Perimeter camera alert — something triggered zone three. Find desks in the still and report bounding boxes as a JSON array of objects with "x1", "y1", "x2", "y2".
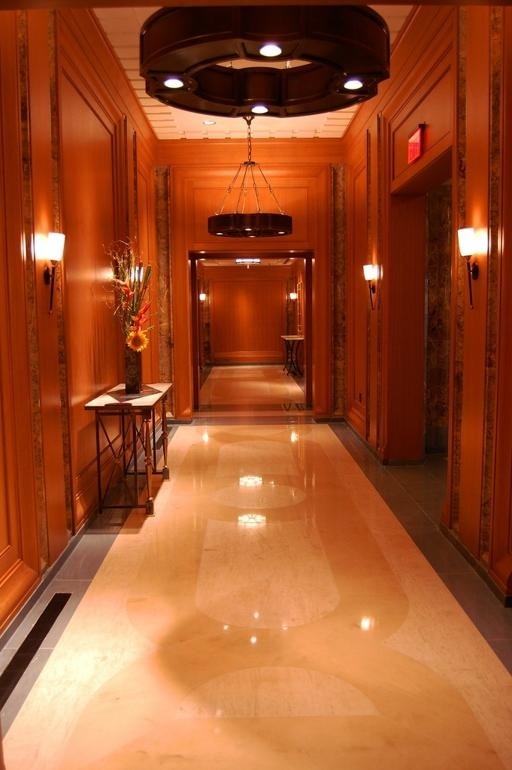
[
  {"x1": 81, "y1": 378, "x2": 176, "y2": 518},
  {"x1": 279, "y1": 334, "x2": 306, "y2": 382}
]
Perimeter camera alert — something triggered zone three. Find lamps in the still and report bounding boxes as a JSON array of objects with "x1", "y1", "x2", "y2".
[
  {"x1": 289, "y1": 291, "x2": 297, "y2": 302},
  {"x1": 452, "y1": 225, "x2": 481, "y2": 309},
  {"x1": 39, "y1": 231, "x2": 67, "y2": 313},
  {"x1": 137, "y1": 3, "x2": 393, "y2": 123},
  {"x1": 361, "y1": 261, "x2": 374, "y2": 305},
  {"x1": 199, "y1": 294, "x2": 207, "y2": 301},
  {"x1": 203, "y1": 111, "x2": 293, "y2": 243}
]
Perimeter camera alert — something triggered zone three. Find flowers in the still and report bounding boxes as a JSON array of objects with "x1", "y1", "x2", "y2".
[{"x1": 104, "y1": 233, "x2": 169, "y2": 353}]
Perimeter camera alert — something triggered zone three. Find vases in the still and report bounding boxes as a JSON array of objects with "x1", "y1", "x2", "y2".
[{"x1": 125, "y1": 347, "x2": 141, "y2": 394}]
